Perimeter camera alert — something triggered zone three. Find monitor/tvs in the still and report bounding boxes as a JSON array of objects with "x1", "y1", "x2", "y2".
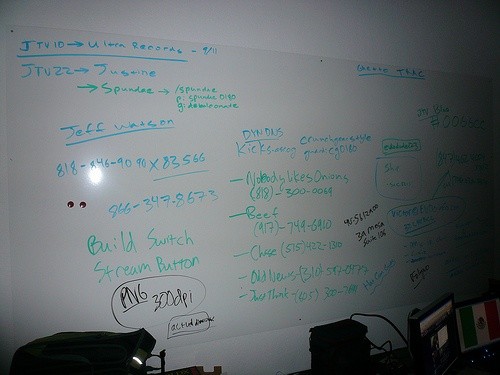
[{"x1": 408, "y1": 292, "x2": 461, "y2": 375}]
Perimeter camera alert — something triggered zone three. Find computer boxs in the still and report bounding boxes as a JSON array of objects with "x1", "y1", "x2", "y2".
[{"x1": 309, "y1": 318, "x2": 370, "y2": 375}]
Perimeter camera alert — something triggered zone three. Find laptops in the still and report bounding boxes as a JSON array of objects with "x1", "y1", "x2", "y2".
[{"x1": 453, "y1": 295, "x2": 500, "y2": 375}]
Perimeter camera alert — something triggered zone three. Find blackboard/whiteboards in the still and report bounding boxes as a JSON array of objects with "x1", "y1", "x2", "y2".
[{"x1": 0, "y1": 24, "x2": 500, "y2": 374}]
